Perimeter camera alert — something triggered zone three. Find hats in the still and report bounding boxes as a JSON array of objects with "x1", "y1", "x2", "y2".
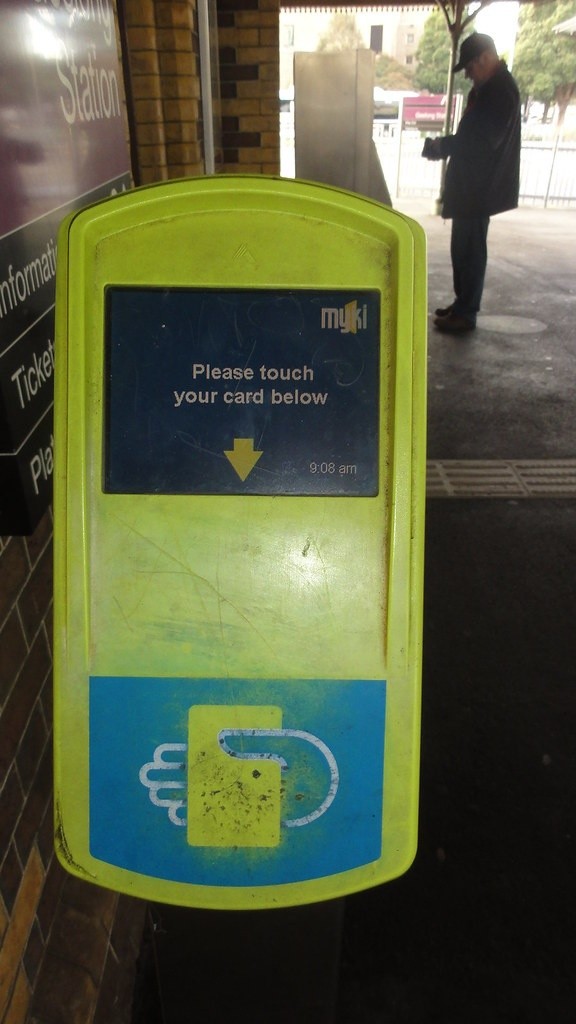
[{"x1": 453, "y1": 33, "x2": 494, "y2": 73}]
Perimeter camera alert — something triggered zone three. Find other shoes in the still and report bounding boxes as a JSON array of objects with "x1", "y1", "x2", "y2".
[
  {"x1": 435, "y1": 311, "x2": 476, "y2": 331},
  {"x1": 435, "y1": 304, "x2": 454, "y2": 316}
]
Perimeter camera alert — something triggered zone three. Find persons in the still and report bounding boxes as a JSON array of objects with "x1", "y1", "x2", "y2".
[{"x1": 421, "y1": 31, "x2": 522, "y2": 332}]
